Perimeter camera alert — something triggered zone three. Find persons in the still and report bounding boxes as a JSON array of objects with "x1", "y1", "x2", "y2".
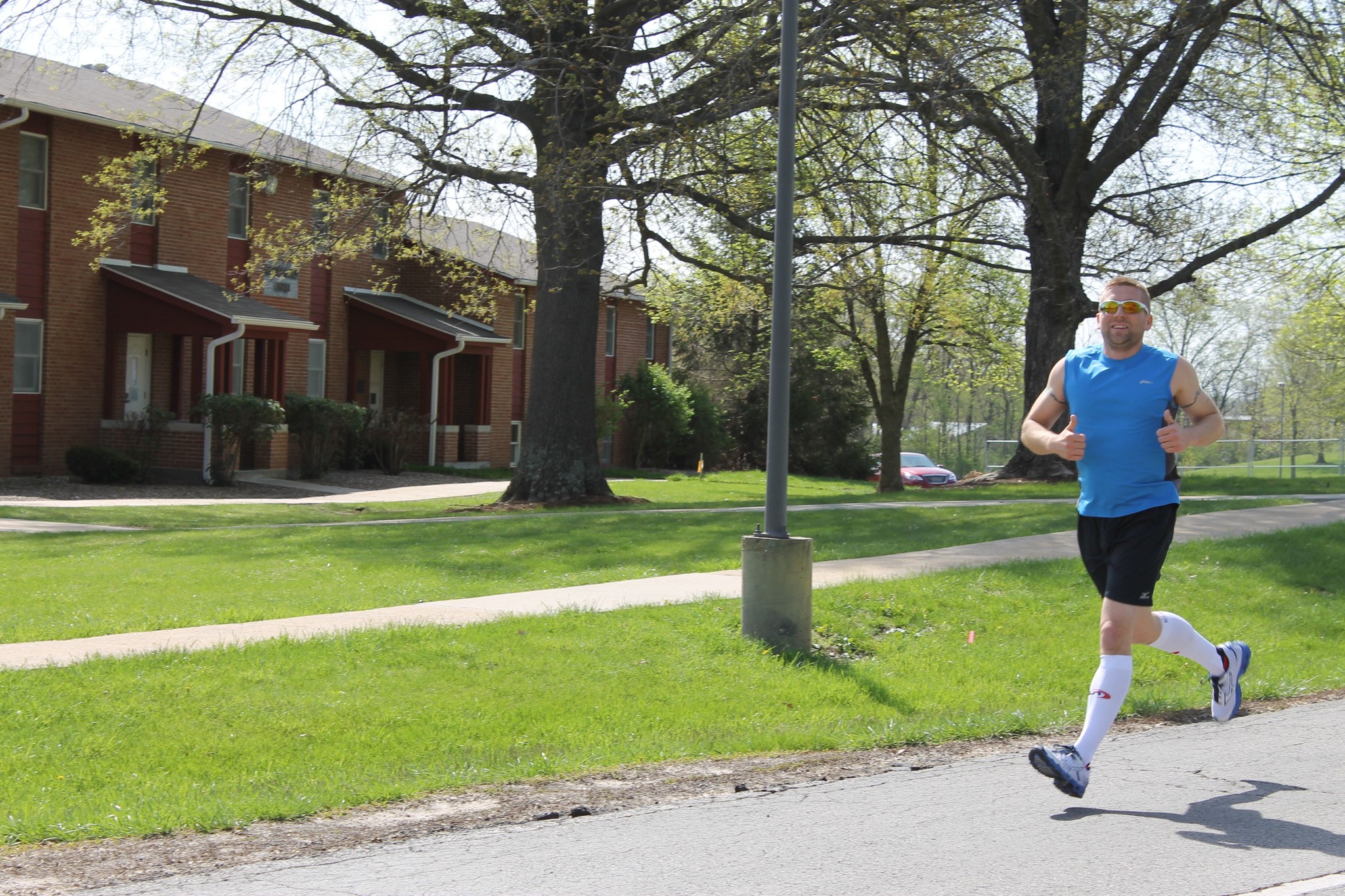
[{"x1": 1016, "y1": 277, "x2": 1253, "y2": 799}]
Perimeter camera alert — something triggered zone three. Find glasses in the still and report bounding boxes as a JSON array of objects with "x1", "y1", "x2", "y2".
[{"x1": 1098, "y1": 299, "x2": 1151, "y2": 316}]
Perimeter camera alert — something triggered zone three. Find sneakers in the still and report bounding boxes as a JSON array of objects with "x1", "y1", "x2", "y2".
[
  {"x1": 1202, "y1": 640, "x2": 1250, "y2": 721},
  {"x1": 1027, "y1": 742, "x2": 1091, "y2": 797}
]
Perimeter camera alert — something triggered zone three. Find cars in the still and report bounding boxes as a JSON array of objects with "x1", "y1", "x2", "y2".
[{"x1": 867, "y1": 452, "x2": 957, "y2": 489}]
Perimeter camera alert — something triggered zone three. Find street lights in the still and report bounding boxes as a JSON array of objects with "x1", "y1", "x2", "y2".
[{"x1": 1277, "y1": 382, "x2": 1285, "y2": 478}]
